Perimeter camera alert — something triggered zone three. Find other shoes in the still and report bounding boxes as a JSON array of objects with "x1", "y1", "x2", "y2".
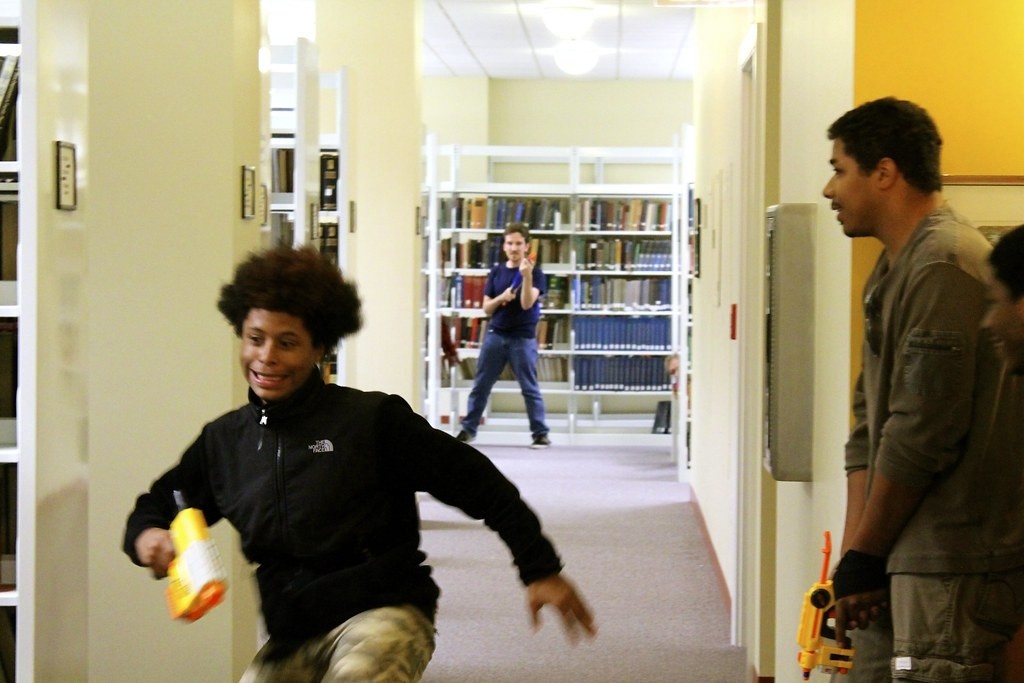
[
  {"x1": 530, "y1": 436, "x2": 551, "y2": 448},
  {"x1": 456, "y1": 429, "x2": 475, "y2": 444}
]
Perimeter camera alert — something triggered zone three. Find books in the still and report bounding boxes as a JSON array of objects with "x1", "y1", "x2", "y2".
[
  {"x1": 271, "y1": 149, "x2": 339, "y2": 375},
  {"x1": 0, "y1": 55, "x2": 19, "y2": 683},
  {"x1": 687, "y1": 183, "x2": 692, "y2": 468},
  {"x1": 439, "y1": 198, "x2": 672, "y2": 392}
]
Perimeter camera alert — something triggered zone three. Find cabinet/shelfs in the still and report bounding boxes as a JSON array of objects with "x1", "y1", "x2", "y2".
[
  {"x1": 319, "y1": 57, "x2": 348, "y2": 385},
  {"x1": 0, "y1": 0, "x2": 90, "y2": 683},
  {"x1": 268, "y1": 37, "x2": 320, "y2": 252},
  {"x1": 417, "y1": 148, "x2": 681, "y2": 440}
]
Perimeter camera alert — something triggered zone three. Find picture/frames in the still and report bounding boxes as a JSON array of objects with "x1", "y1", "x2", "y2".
[
  {"x1": 241, "y1": 165, "x2": 257, "y2": 218},
  {"x1": 694, "y1": 233, "x2": 701, "y2": 279},
  {"x1": 350, "y1": 199, "x2": 358, "y2": 232},
  {"x1": 56, "y1": 141, "x2": 78, "y2": 211},
  {"x1": 310, "y1": 203, "x2": 319, "y2": 241},
  {"x1": 259, "y1": 185, "x2": 268, "y2": 226},
  {"x1": 422, "y1": 216, "x2": 429, "y2": 239},
  {"x1": 694, "y1": 198, "x2": 701, "y2": 224},
  {"x1": 416, "y1": 207, "x2": 420, "y2": 235},
  {"x1": 436, "y1": 219, "x2": 440, "y2": 241}
]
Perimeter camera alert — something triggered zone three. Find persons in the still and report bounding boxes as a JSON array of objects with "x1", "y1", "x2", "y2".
[
  {"x1": 455, "y1": 224, "x2": 552, "y2": 449},
  {"x1": 123, "y1": 242, "x2": 597, "y2": 683},
  {"x1": 822, "y1": 96, "x2": 1024, "y2": 683},
  {"x1": 665, "y1": 353, "x2": 680, "y2": 397}
]
